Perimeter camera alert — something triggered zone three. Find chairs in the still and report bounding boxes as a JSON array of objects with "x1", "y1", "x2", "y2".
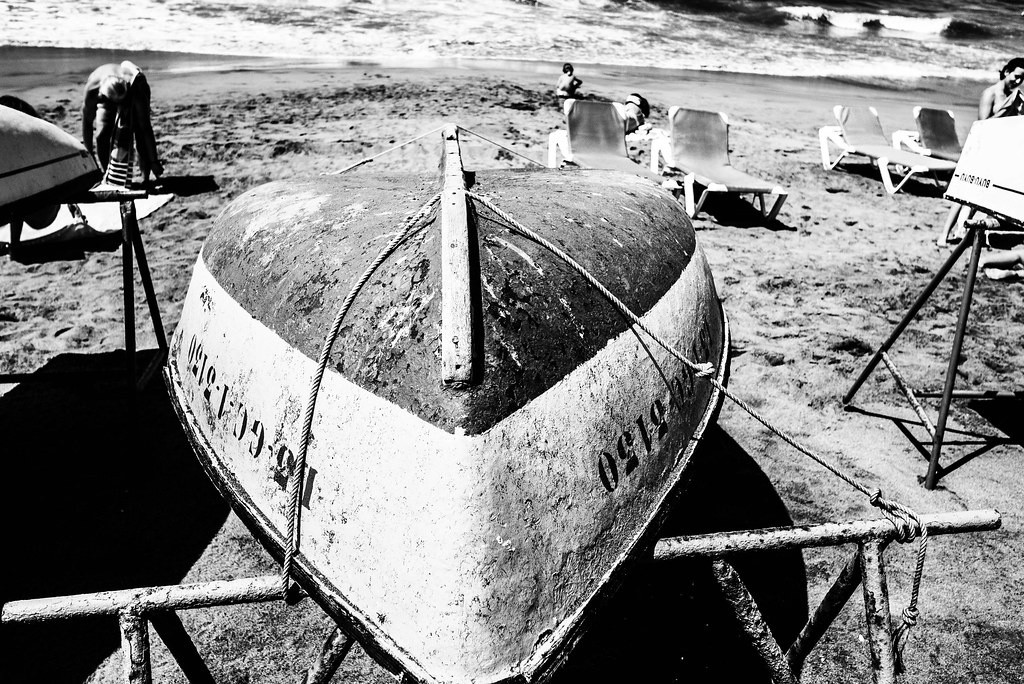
[
  {"x1": 819, "y1": 103, "x2": 957, "y2": 196},
  {"x1": 547, "y1": 98, "x2": 683, "y2": 200},
  {"x1": 891, "y1": 106, "x2": 963, "y2": 177},
  {"x1": 650, "y1": 104, "x2": 788, "y2": 223}
]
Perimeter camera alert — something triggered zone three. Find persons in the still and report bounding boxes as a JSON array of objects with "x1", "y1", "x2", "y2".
[
  {"x1": 557, "y1": 62, "x2": 582, "y2": 96},
  {"x1": 82, "y1": 61, "x2": 164, "y2": 189},
  {"x1": 937, "y1": 57, "x2": 1024, "y2": 251}
]
